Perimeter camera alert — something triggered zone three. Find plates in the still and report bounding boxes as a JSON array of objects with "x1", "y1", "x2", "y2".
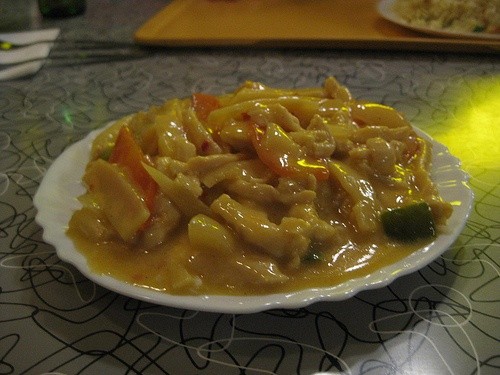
[
  {"x1": 33, "y1": 114, "x2": 475, "y2": 313},
  {"x1": 379, "y1": 0, "x2": 500, "y2": 39}
]
